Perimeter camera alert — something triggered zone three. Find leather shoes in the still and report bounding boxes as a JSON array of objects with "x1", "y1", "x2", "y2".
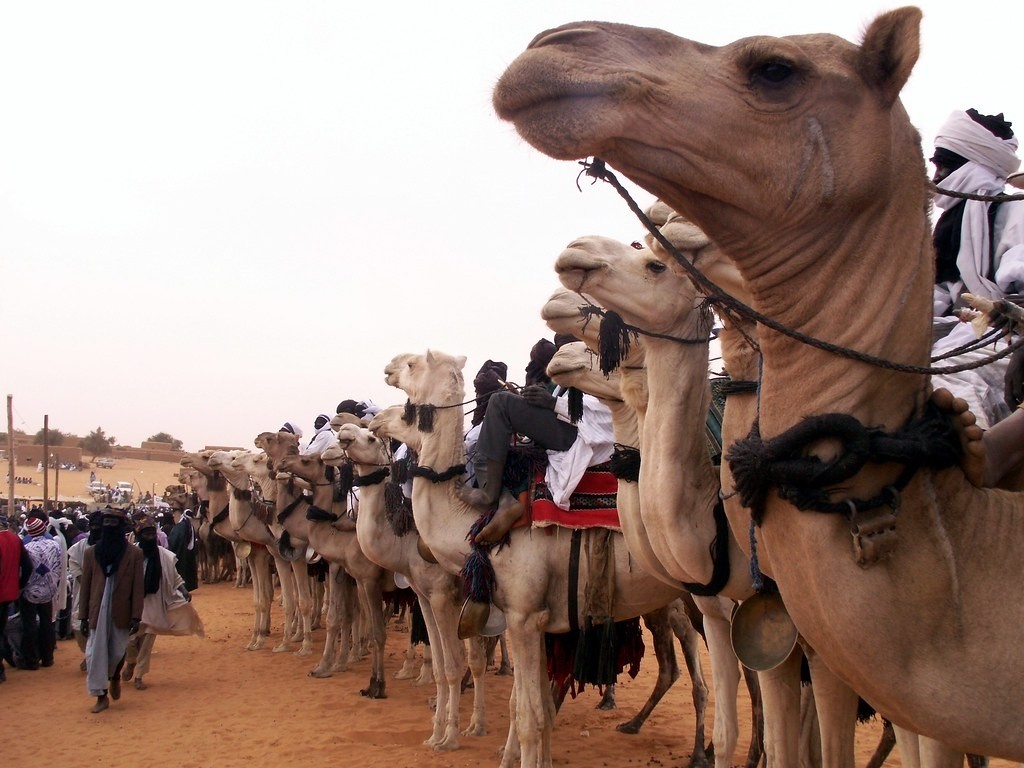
[{"x1": 17, "y1": 655, "x2": 54, "y2": 670}]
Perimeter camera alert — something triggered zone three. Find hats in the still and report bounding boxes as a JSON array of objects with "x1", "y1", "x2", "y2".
[
  {"x1": 929, "y1": 108, "x2": 1015, "y2": 164},
  {"x1": 27, "y1": 517, "x2": 47, "y2": 537},
  {"x1": 101, "y1": 506, "x2": 125, "y2": 519},
  {"x1": 474, "y1": 360, "x2": 507, "y2": 389},
  {"x1": 337, "y1": 400, "x2": 367, "y2": 419},
  {"x1": 554, "y1": 332, "x2": 581, "y2": 350},
  {"x1": 530, "y1": 338, "x2": 558, "y2": 368}
]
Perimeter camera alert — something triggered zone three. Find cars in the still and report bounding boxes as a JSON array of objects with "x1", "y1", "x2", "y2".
[
  {"x1": 86, "y1": 481, "x2": 107, "y2": 497},
  {"x1": 115, "y1": 481, "x2": 134, "y2": 497}
]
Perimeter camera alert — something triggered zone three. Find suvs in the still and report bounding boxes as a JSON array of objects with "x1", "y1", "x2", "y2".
[{"x1": 95, "y1": 458, "x2": 114, "y2": 469}]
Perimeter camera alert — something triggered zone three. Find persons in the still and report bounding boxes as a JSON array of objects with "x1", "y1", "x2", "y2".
[
  {"x1": 281, "y1": 423, "x2": 301, "y2": 444},
  {"x1": 1, "y1": 512, "x2": 33, "y2": 683},
  {"x1": 19, "y1": 519, "x2": 60, "y2": 669},
  {"x1": 1, "y1": 486, "x2": 177, "y2": 549},
  {"x1": 6, "y1": 474, "x2": 32, "y2": 483},
  {"x1": 79, "y1": 507, "x2": 145, "y2": 713},
  {"x1": 89, "y1": 470, "x2": 95, "y2": 482},
  {"x1": 45, "y1": 517, "x2": 67, "y2": 611},
  {"x1": 341, "y1": 400, "x2": 396, "y2": 517},
  {"x1": 169, "y1": 507, "x2": 199, "y2": 592},
  {"x1": 503, "y1": 336, "x2": 558, "y2": 394},
  {"x1": 303, "y1": 412, "x2": 340, "y2": 504},
  {"x1": 67, "y1": 511, "x2": 104, "y2": 670},
  {"x1": 37, "y1": 459, "x2": 76, "y2": 474},
  {"x1": 454, "y1": 327, "x2": 621, "y2": 546},
  {"x1": 465, "y1": 359, "x2": 507, "y2": 443},
  {"x1": 924, "y1": 106, "x2": 1023, "y2": 435},
  {"x1": 22, "y1": 509, "x2": 53, "y2": 544},
  {"x1": 934, "y1": 337, "x2": 1024, "y2": 490},
  {"x1": 121, "y1": 516, "x2": 205, "y2": 691}
]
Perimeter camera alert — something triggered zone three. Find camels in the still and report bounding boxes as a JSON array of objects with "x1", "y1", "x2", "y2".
[{"x1": 162, "y1": 7, "x2": 1023, "y2": 767}]
